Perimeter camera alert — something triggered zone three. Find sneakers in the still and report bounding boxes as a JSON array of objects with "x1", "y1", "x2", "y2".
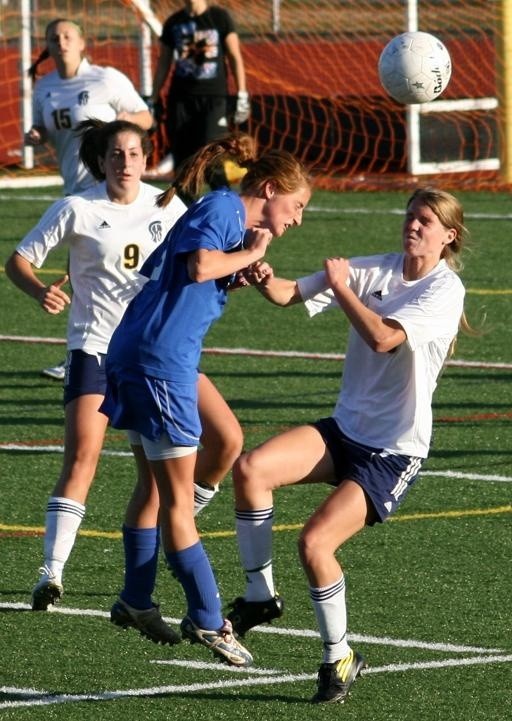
[
  {"x1": 109, "y1": 598, "x2": 181, "y2": 645},
  {"x1": 31, "y1": 566, "x2": 64, "y2": 611},
  {"x1": 312, "y1": 650, "x2": 364, "y2": 703},
  {"x1": 182, "y1": 616, "x2": 253, "y2": 666},
  {"x1": 228, "y1": 592, "x2": 282, "y2": 637}
]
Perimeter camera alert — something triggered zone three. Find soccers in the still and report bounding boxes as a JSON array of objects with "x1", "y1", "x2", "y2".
[{"x1": 378, "y1": 31, "x2": 452, "y2": 105}]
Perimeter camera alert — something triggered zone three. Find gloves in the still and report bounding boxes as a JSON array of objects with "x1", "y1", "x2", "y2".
[{"x1": 234, "y1": 92, "x2": 250, "y2": 124}]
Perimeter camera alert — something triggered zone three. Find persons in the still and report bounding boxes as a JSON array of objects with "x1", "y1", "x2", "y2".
[
  {"x1": 98, "y1": 133, "x2": 310, "y2": 668},
  {"x1": 226, "y1": 188, "x2": 489, "y2": 706},
  {"x1": 4, "y1": 119, "x2": 245, "y2": 611},
  {"x1": 151, "y1": 0, "x2": 252, "y2": 208},
  {"x1": 24, "y1": 18, "x2": 155, "y2": 381}
]
